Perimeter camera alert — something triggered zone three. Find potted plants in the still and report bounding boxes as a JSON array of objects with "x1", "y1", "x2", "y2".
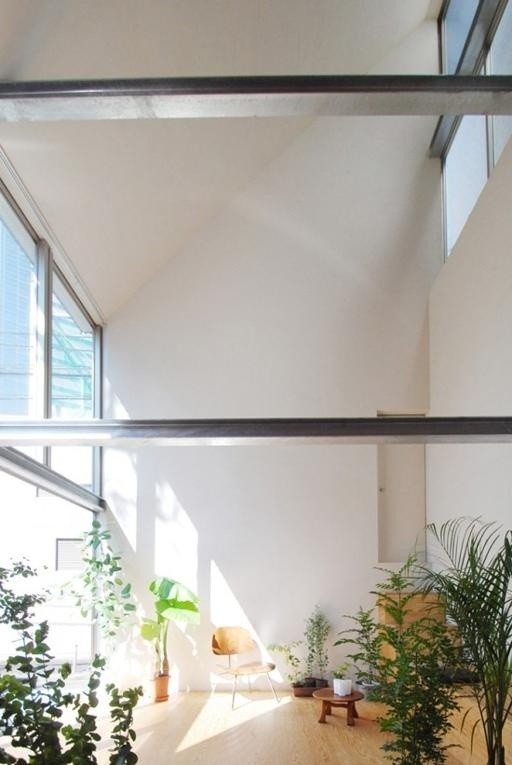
[
  {"x1": 266, "y1": 599, "x2": 389, "y2": 704},
  {"x1": 140, "y1": 576, "x2": 201, "y2": 704}
]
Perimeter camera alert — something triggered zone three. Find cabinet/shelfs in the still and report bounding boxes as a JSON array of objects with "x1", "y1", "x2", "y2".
[{"x1": 377, "y1": 591, "x2": 465, "y2": 687}]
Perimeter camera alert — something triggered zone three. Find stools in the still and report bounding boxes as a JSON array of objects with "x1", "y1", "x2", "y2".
[{"x1": 312, "y1": 688, "x2": 364, "y2": 727}]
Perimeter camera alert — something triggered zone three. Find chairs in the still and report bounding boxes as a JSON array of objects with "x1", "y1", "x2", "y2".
[{"x1": 204, "y1": 624, "x2": 280, "y2": 713}]
